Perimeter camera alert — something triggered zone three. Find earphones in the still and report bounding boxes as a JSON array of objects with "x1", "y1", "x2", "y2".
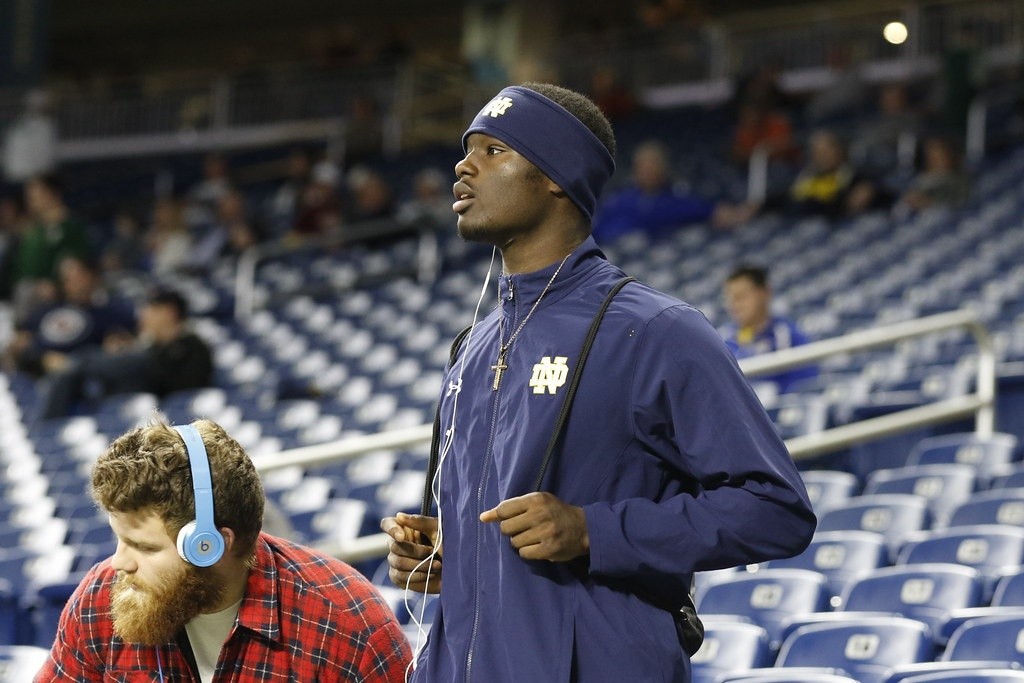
[{"x1": 413, "y1": 648, "x2": 419, "y2": 670}]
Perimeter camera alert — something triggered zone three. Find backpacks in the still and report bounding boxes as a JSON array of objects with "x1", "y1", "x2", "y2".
[{"x1": 672, "y1": 573, "x2": 705, "y2": 658}]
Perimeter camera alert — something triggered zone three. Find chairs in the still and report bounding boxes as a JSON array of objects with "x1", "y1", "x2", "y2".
[{"x1": 0, "y1": 160, "x2": 1024, "y2": 683}]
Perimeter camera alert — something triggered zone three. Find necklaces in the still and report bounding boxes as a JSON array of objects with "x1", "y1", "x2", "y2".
[{"x1": 491, "y1": 254, "x2": 574, "y2": 391}]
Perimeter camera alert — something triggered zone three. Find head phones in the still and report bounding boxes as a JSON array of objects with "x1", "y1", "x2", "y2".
[{"x1": 170, "y1": 425, "x2": 225, "y2": 566}]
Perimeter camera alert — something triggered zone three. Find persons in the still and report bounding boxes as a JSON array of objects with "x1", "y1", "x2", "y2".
[
  {"x1": 29, "y1": 419, "x2": 414, "y2": 683},
  {"x1": 379, "y1": 84, "x2": 818, "y2": 682},
  {"x1": 716, "y1": 267, "x2": 819, "y2": 441},
  {"x1": 0, "y1": 49, "x2": 504, "y2": 421},
  {"x1": 591, "y1": 29, "x2": 1007, "y2": 244}
]
